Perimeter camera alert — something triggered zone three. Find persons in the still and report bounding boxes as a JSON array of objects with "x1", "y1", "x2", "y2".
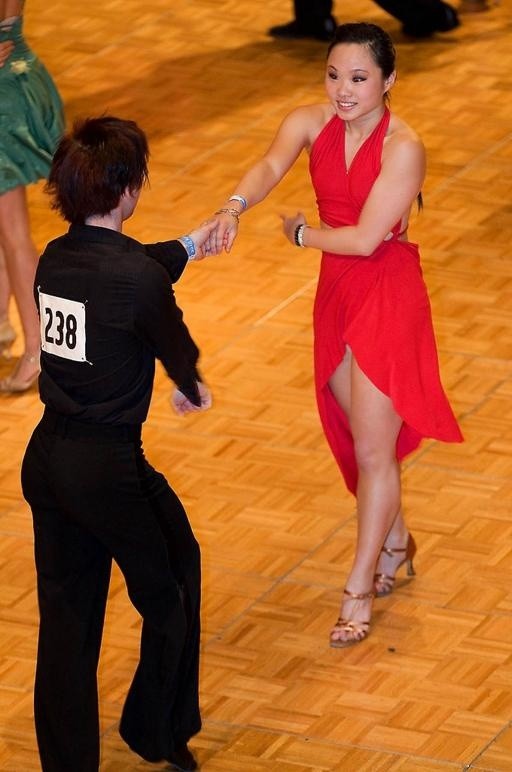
[
  {"x1": 1, "y1": 0, "x2": 62, "y2": 392},
  {"x1": 264, "y1": 0, "x2": 460, "y2": 36},
  {"x1": 205, "y1": 23, "x2": 462, "y2": 649},
  {"x1": 214, "y1": 208, "x2": 241, "y2": 221},
  {"x1": 227, "y1": 195, "x2": 248, "y2": 206},
  {"x1": 19, "y1": 118, "x2": 225, "y2": 768}
]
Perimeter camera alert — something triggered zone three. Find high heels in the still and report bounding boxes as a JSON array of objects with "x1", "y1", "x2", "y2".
[
  {"x1": 1, "y1": 351, "x2": 42, "y2": 393},
  {"x1": 374, "y1": 532, "x2": 417, "y2": 597},
  {"x1": 0, "y1": 327, "x2": 17, "y2": 355},
  {"x1": 330, "y1": 587, "x2": 375, "y2": 647}
]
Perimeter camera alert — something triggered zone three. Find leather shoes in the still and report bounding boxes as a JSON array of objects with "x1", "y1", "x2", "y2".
[
  {"x1": 267, "y1": 16, "x2": 337, "y2": 43},
  {"x1": 131, "y1": 737, "x2": 198, "y2": 772},
  {"x1": 399, "y1": 3, "x2": 461, "y2": 40}
]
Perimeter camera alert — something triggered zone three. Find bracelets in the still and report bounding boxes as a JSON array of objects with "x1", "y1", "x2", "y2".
[
  {"x1": 294, "y1": 223, "x2": 305, "y2": 248},
  {"x1": 175, "y1": 235, "x2": 194, "y2": 255}
]
